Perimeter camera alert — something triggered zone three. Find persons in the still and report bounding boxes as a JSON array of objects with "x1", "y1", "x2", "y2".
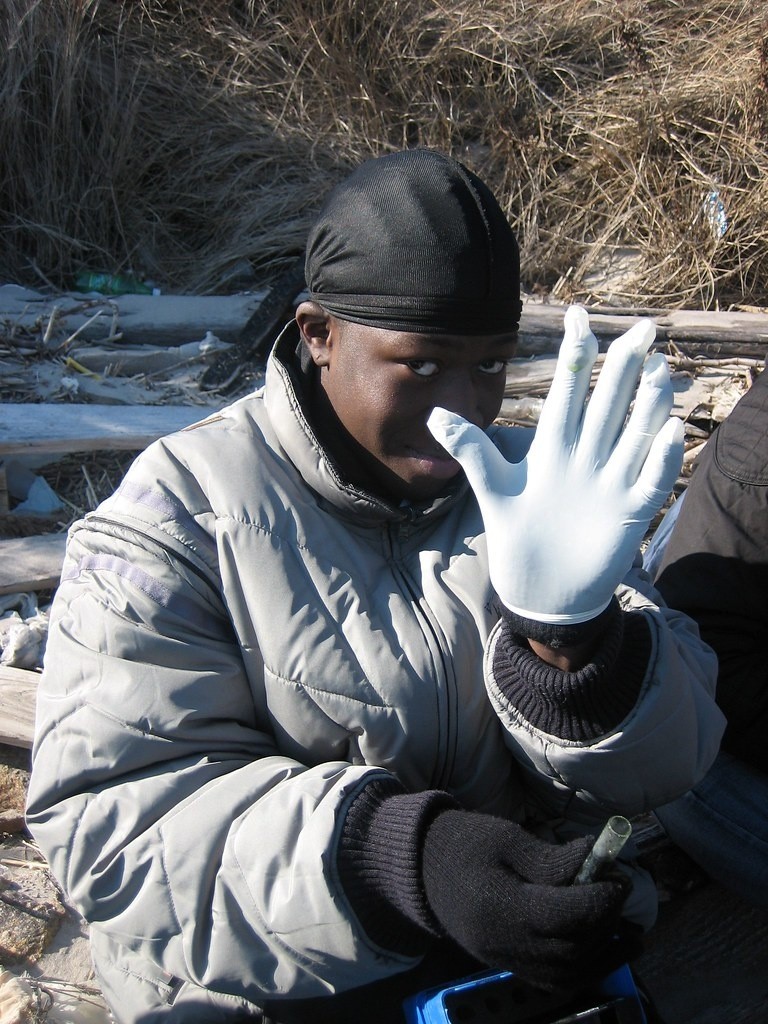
[
  {"x1": 23, "y1": 151, "x2": 726, "y2": 1024},
  {"x1": 641, "y1": 352, "x2": 768, "y2": 903}
]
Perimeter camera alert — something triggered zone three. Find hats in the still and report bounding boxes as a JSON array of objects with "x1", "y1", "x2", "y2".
[{"x1": 304, "y1": 152, "x2": 521, "y2": 334}]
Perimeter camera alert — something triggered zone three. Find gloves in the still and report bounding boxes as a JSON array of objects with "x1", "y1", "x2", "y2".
[
  {"x1": 426, "y1": 304, "x2": 686, "y2": 652},
  {"x1": 420, "y1": 809, "x2": 634, "y2": 987}
]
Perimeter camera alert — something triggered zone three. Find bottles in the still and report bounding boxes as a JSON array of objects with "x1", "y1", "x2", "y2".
[
  {"x1": 78, "y1": 272, "x2": 162, "y2": 298},
  {"x1": 706, "y1": 191, "x2": 729, "y2": 237}
]
